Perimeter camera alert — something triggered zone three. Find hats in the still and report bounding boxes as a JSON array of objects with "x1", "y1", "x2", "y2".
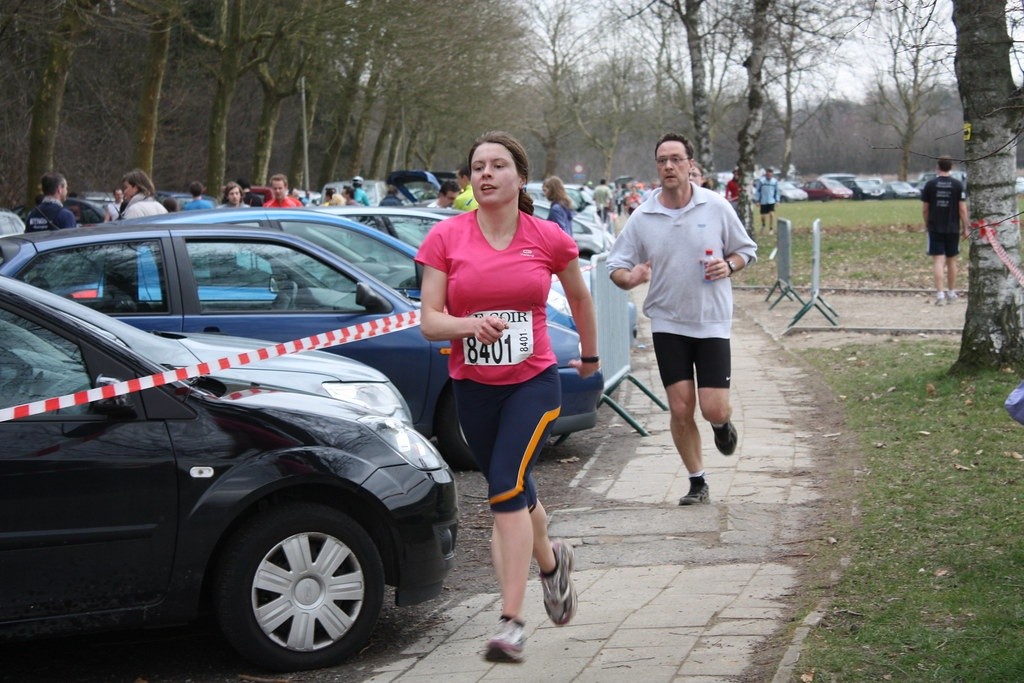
[{"x1": 351, "y1": 176, "x2": 364, "y2": 184}]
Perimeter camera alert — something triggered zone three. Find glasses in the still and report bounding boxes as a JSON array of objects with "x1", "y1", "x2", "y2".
[{"x1": 655, "y1": 156, "x2": 691, "y2": 165}]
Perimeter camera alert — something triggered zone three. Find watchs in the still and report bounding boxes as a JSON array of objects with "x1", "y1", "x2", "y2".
[{"x1": 727, "y1": 261, "x2": 736, "y2": 276}]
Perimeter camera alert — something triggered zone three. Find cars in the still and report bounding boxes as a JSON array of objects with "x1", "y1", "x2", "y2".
[
  {"x1": 0, "y1": 208, "x2": 604, "y2": 473},
  {"x1": 710, "y1": 165, "x2": 970, "y2": 199},
  {"x1": 0, "y1": 276, "x2": 462, "y2": 668},
  {"x1": 15, "y1": 170, "x2": 637, "y2": 345}
]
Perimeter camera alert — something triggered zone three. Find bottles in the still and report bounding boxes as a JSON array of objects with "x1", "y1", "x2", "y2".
[{"x1": 700, "y1": 250, "x2": 716, "y2": 284}]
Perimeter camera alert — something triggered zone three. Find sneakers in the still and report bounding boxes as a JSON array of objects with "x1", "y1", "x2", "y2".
[
  {"x1": 678, "y1": 473, "x2": 710, "y2": 506},
  {"x1": 934, "y1": 297, "x2": 948, "y2": 307},
  {"x1": 715, "y1": 423, "x2": 738, "y2": 457},
  {"x1": 486, "y1": 614, "x2": 526, "y2": 664},
  {"x1": 948, "y1": 296, "x2": 965, "y2": 306},
  {"x1": 540, "y1": 541, "x2": 578, "y2": 625}
]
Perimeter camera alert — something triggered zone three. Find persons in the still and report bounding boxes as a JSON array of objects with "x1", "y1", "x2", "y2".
[
  {"x1": 606, "y1": 132, "x2": 758, "y2": 506},
  {"x1": 182, "y1": 181, "x2": 214, "y2": 210},
  {"x1": 452, "y1": 163, "x2": 479, "y2": 211},
  {"x1": 754, "y1": 166, "x2": 780, "y2": 235},
  {"x1": 921, "y1": 156, "x2": 969, "y2": 307},
  {"x1": 414, "y1": 131, "x2": 600, "y2": 663},
  {"x1": 290, "y1": 176, "x2": 370, "y2": 206},
  {"x1": 379, "y1": 187, "x2": 403, "y2": 206},
  {"x1": 542, "y1": 176, "x2": 573, "y2": 237},
  {"x1": 689, "y1": 161, "x2": 706, "y2": 188},
  {"x1": 236, "y1": 178, "x2": 262, "y2": 207},
  {"x1": 593, "y1": 179, "x2": 660, "y2": 235},
  {"x1": 427, "y1": 181, "x2": 461, "y2": 210},
  {"x1": 104, "y1": 170, "x2": 178, "y2": 222},
  {"x1": 216, "y1": 181, "x2": 250, "y2": 208},
  {"x1": 262, "y1": 174, "x2": 303, "y2": 208},
  {"x1": 24, "y1": 171, "x2": 76, "y2": 232},
  {"x1": 725, "y1": 166, "x2": 741, "y2": 210}
]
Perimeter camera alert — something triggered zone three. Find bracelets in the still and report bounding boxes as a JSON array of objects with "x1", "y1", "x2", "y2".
[{"x1": 580, "y1": 356, "x2": 598, "y2": 362}]
